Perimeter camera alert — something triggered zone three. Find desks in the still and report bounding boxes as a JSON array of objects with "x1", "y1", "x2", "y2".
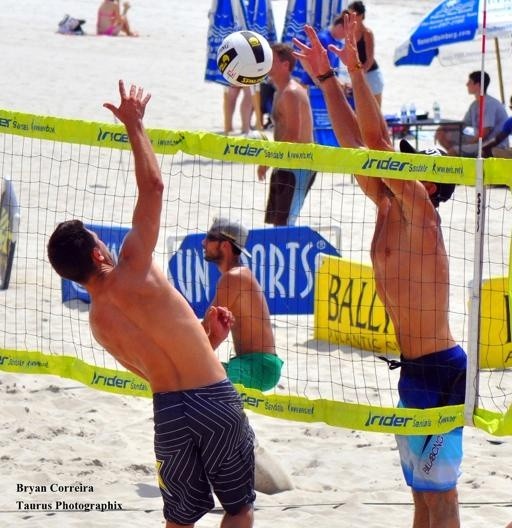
[{"x1": 388, "y1": 117, "x2": 465, "y2": 156}]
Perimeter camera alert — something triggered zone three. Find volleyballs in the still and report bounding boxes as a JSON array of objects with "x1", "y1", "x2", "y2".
[{"x1": 216, "y1": 29, "x2": 273, "y2": 86}]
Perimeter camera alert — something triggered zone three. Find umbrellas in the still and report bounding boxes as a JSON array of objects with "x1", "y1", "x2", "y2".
[
  {"x1": 204, "y1": 0, "x2": 277, "y2": 88},
  {"x1": 282, "y1": 0, "x2": 347, "y2": 85},
  {"x1": 394, "y1": 1, "x2": 511, "y2": 105}
]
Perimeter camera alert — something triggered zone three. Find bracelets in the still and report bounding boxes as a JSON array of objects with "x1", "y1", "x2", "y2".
[
  {"x1": 347, "y1": 61, "x2": 362, "y2": 72},
  {"x1": 316, "y1": 69, "x2": 335, "y2": 82}
]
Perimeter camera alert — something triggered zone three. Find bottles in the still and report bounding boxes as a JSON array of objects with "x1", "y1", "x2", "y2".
[
  {"x1": 400, "y1": 104, "x2": 407, "y2": 123},
  {"x1": 409, "y1": 103, "x2": 416, "y2": 122},
  {"x1": 464, "y1": 127, "x2": 480, "y2": 137},
  {"x1": 432, "y1": 101, "x2": 440, "y2": 122}
]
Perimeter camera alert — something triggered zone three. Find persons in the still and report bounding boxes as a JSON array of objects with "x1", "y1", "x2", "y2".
[
  {"x1": 258, "y1": 42, "x2": 319, "y2": 228},
  {"x1": 50, "y1": 77, "x2": 255, "y2": 527},
  {"x1": 481, "y1": 96, "x2": 511, "y2": 159},
  {"x1": 290, "y1": 12, "x2": 468, "y2": 528},
  {"x1": 219, "y1": 79, "x2": 253, "y2": 136},
  {"x1": 434, "y1": 71, "x2": 508, "y2": 156},
  {"x1": 347, "y1": 1, "x2": 384, "y2": 110},
  {"x1": 301, "y1": 11, "x2": 345, "y2": 85},
  {"x1": 198, "y1": 217, "x2": 283, "y2": 391},
  {"x1": 96, "y1": 0, "x2": 138, "y2": 37}
]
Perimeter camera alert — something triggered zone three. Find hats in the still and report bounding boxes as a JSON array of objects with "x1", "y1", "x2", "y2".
[
  {"x1": 400, "y1": 138, "x2": 456, "y2": 202},
  {"x1": 209, "y1": 216, "x2": 252, "y2": 259}
]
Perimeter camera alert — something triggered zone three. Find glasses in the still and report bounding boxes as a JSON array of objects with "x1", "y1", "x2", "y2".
[{"x1": 207, "y1": 234, "x2": 225, "y2": 242}]
